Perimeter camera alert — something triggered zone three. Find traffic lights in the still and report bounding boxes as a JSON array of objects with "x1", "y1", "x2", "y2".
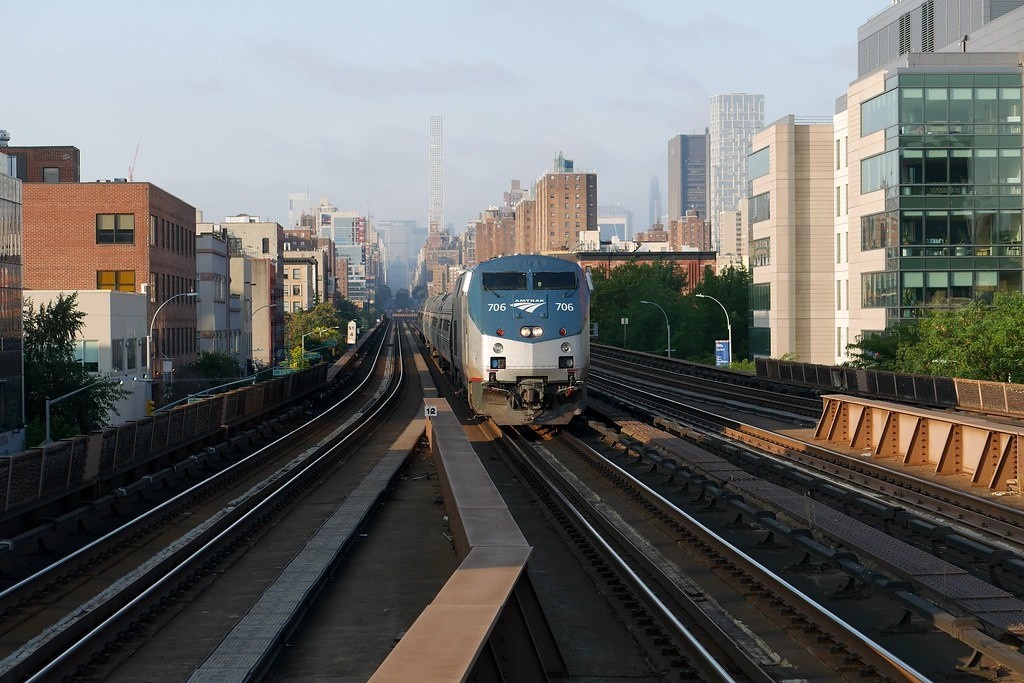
[{"x1": 145, "y1": 399, "x2": 156, "y2": 416}]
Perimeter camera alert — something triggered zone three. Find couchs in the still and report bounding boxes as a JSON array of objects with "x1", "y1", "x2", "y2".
[{"x1": 922, "y1": 239, "x2": 944, "y2": 256}]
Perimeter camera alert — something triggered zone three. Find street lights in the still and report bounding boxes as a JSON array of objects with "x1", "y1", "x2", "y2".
[
  {"x1": 244, "y1": 304, "x2": 278, "y2": 378},
  {"x1": 146, "y1": 293, "x2": 198, "y2": 405},
  {"x1": 320, "y1": 326, "x2": 339, "y2": 343},
  {"x1": 695, "y1": 294, "x2": 732, "y2": 370},
  {"x1": 301, "y1": 328, "x2": 319, "y2": 367},
  {"x1": 45, "y1": 375, "x2": 136, "y2": 445},
  {"x1": 641, "y1": 300, "x2": 671, "y2": 358}
]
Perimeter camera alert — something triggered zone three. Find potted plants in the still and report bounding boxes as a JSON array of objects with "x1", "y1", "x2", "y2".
[
  {"x1": 954, "y1": 230, "x2": 967, "y2": 256},
  {"x1": 902, "y1": 229, "x2": 911, "y2": 245},
  {"x1": 959, "y1": 176, "x2": 969, "y2": 194},
  {"x1": 901, "y1": 174, "x2": 913, "y2": 196},
  {"x1": 903, "y1": 288, "x2": 917, "y2": 317}
]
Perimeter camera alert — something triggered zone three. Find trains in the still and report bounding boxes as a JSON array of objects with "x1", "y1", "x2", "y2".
[{"x1": 417, "y1": 254, "x2": 595, "y2": 427}]
